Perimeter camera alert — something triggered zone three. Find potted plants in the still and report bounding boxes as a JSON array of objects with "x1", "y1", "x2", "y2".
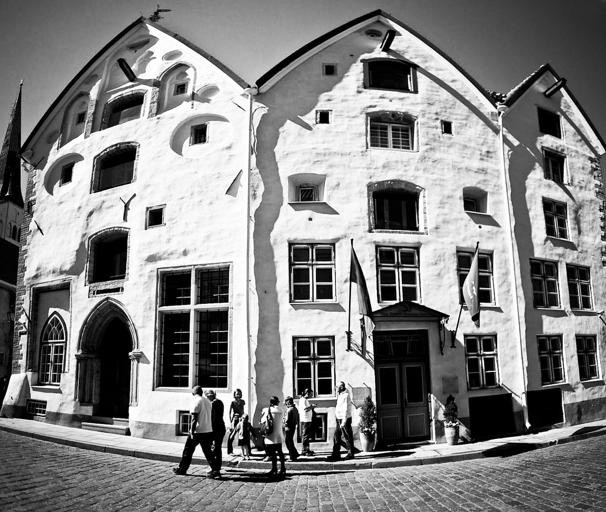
[
  {"x1": 358, "y1": 396, "x2": 377, "y2": 452},
  {"x1": 443, "y1": 393, "x2": 459, "y2": 445}
]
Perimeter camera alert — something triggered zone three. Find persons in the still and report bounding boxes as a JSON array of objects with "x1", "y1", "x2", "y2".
[{"x1": 171, "y1": 381, "x2": 355, "y2": 479}]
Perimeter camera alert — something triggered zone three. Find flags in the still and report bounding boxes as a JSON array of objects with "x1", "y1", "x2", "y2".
[
  {"x1": 350, "y1": 239, "x2": 375, "y2": 337},
  {"x1": 462, "y1": 250, "x2": 480, "y2": 329}
]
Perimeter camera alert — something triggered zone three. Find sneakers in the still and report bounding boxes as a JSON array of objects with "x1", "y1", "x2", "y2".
[
  {"x1": 228, "y1": 450, "x2": 355, "y2": 476},
  {"x1": 173, "y1": 467, "x2": 222, "y2": 479}
]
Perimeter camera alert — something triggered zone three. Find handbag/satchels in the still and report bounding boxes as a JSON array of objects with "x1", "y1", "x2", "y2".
[{"x1": 258, "y1": 418, "x2": 273, "y2": 437}]
